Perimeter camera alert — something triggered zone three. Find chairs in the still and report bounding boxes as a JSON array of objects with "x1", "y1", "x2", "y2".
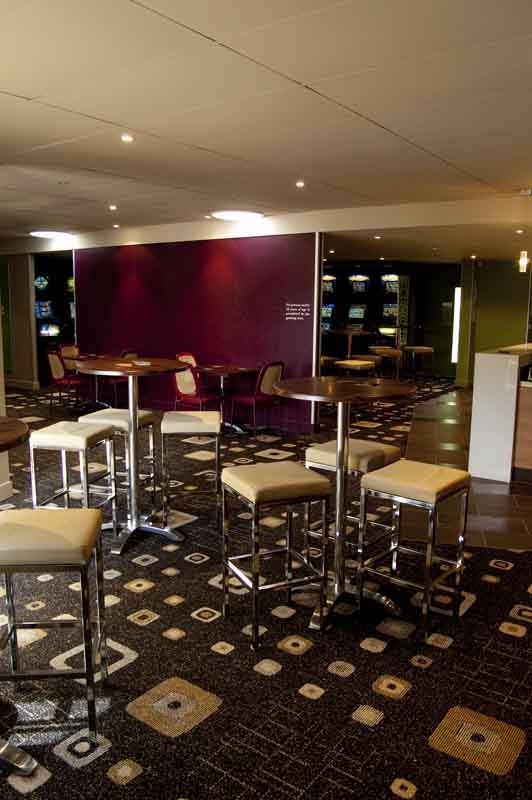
[{"x1": 46, "y1": 345, "x2": 286, "y2": 439}]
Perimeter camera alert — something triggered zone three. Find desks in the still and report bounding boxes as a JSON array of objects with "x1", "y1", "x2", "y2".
[
  {"x1": 73, "y1": 356, "x2": 189, "y2": 554},
  {"x1": 272, "y1": 374, "x2": 419, "y2": 631},
  {"x1": 327, "y1": 329, "x2": 376, "y2": 377}
]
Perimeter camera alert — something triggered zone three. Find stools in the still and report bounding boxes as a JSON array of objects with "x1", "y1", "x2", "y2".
[
  {"x1": 222, "y1": 461, "x2": 330, "y2": 648},
  {"x1": 29, "y1": 420, "x2": 116, "y2": 536},
  {"x1": 0, "y1": 507, "x2": 107, "y2": 749},
  {"x1": 304, "y1": 438, "x2": 400, "y2": 571},
  {"x1": 355, "y1": 458, "x2": 471, "y2": 635},
  {"x1": 160, "y1": 409, "x2": 222, "y2": 529},
  {"x1": 79, "y1": 406, "x2": 153, "y2": 518},
  {"x1": 320, "y1": 343, "x2": 436, "y2": 382}
]
